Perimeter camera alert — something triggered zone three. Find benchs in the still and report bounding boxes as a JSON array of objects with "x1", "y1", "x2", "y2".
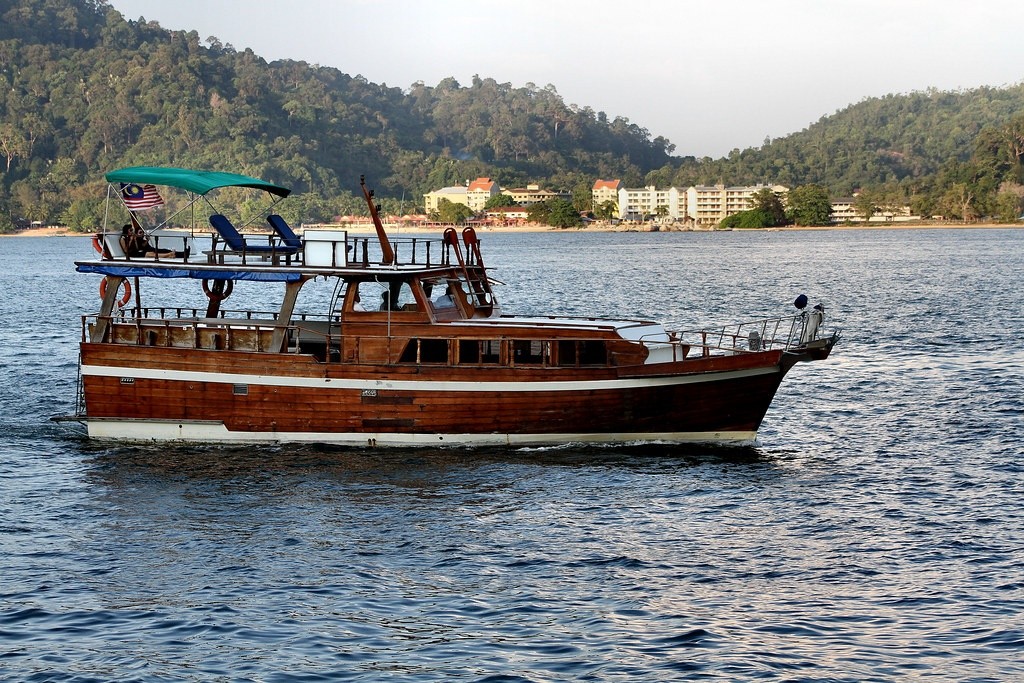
[
  {"x1": 102, "y1": 230, "x2": 194, "y2": 262},
  {"x1": 88, "y1": 316, "x2": 340, "y2": 352}
]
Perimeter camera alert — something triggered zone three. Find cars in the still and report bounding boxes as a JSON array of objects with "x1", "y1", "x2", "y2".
[{"x1": 578, "y1": 215, "x2": 598, "y2": 225}]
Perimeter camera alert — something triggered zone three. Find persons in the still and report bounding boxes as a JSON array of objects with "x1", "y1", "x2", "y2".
[
  {"x1": 380, "y1": 291, "x2": 388, "y2": 310},
  {"x1": 434, "y1": 287, "x2": 454, "y2": 309},
  {"x1": 120, "y1": 224, "x2": 190, "y2": 258}
]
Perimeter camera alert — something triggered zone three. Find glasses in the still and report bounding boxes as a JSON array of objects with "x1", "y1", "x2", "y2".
[
  {"x1": 139, "y1": 231, "x2": 145, "y2": 233},
  {"x1": 128, "y1": 229, "x2": 134, "y2": 231}
]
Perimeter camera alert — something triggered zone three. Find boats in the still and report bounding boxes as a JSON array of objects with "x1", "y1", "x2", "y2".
[{"x1": 50, "y1": 175, "x2": 842, "y2": 443}]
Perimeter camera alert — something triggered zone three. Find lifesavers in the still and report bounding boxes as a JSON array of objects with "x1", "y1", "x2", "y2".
[
  {"x1": 201, "y1": 278, "x2": 234, "y2": 301},
  {"x1": 100, "y1": 275, "x2": 132, "y2": 307},
  {"x1": 92, "y1": 234, "x2": 110, "y2": 258}
]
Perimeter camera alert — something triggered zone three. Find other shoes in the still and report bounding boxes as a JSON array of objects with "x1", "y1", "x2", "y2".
[{"x1": 186, "y1": 246, "x2": 190, "y2": 257}]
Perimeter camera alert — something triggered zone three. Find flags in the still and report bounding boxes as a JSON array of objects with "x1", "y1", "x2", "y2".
[{"x1": 121, "y1": 183, "x2": 163, "y2": 210}]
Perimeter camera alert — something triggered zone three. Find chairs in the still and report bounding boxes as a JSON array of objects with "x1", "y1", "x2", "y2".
[
  {"x1": 209, "y1": 214, "x2": 297, "y2": 253},
  {"x1": 266, "y1": 214, "x2": 353, "y2": 251}
]
